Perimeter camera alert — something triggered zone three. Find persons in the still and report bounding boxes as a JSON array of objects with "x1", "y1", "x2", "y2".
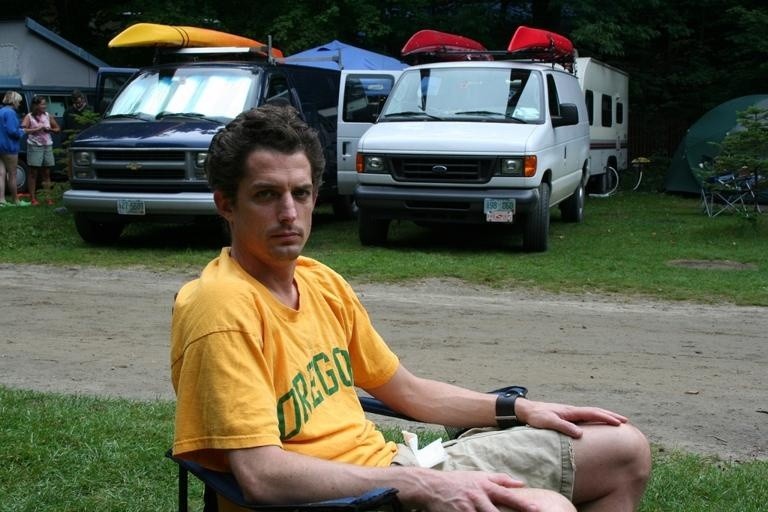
[
  {"x1": 0, "y1": 89, "x2": 32, "y2": 207},
  {"x1": 21, "y1": 96, "x2": 62, "y2": 205},
  {"x1": 63, "y1": 90, "x2": 92, "y2": 179},
  {"x1": 168, "y1": 103, "x2": 653, "y2": 512}
]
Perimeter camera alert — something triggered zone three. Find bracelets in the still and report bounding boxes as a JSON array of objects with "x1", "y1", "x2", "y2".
[{"x1": 493, "y1": 389, "x2": 525, "y2": 429}]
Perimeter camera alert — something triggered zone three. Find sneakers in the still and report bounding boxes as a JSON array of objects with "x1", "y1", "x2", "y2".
[
  {"x1": 0, "y1": 201, "x2": 16, "y2": 207},
  {"x1": 31, "y1": 199, "x2": 38, "y2": 205},
  {"x1": 14, "y1": 200, "x2": 31, "y2": 207}
]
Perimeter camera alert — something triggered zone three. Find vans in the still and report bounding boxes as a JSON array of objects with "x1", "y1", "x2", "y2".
[
  {"x1": 336, "y1": 65, "x2": 591, "y2": 254},
  {"x1": 0, "y1": 77, "x2": 118, "y2": 195},
  {"x1": 62, "y1": 58, "x2": 379, "y2": 249}
]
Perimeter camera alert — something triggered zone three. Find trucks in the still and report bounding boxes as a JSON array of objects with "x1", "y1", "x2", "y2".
[{"x1": 512, "y1": 56, "x2": 634, "y2": 199}]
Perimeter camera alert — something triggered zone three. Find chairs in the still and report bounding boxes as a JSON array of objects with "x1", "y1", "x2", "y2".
[{"x1": 165, "y1": 396, "x2": 424, "y2": 511}]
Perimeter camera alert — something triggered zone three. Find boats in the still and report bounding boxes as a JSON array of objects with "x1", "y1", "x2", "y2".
[
  {"x1": 400, "y1": 28, "x2": 493, "y2": 62},
  {"x1": 507, "y1": 24, "x2": 573, "y2": 57},
  {"x1": 107, "y1": 23, "x2": 287, "y2": 64}
]
